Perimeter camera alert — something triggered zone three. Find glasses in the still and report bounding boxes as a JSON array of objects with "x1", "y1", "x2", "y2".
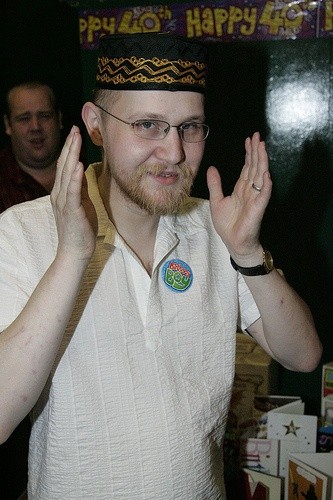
[{"x1": 93, "y1": 103, "x2": 211, "y2": 145}]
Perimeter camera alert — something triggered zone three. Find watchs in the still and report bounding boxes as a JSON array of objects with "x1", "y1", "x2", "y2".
[{"x1": 230, "y1": 245, "x2": 273, "y2": 276}]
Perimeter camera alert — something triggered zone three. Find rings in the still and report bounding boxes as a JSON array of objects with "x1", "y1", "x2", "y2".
[{"x1": 252, "y1": 183, "x2": 262, "y2": 192}]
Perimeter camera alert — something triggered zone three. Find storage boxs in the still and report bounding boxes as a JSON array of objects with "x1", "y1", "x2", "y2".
[{"x1": 224, "y1": 333, "x2": 282, "y2": 442}]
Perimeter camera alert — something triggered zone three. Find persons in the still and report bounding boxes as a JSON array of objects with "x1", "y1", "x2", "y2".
[
  {"x1": 0, "y1": 79, "x2": 62, "y2": 500},
  {"x1": 1, "y1": 32, "x2": 324, "y2": 500}
]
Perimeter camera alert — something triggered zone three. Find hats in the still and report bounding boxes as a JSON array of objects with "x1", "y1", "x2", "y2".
[{"x1": 95, "y1": 32, "x2": 207, "y2": 93}]
